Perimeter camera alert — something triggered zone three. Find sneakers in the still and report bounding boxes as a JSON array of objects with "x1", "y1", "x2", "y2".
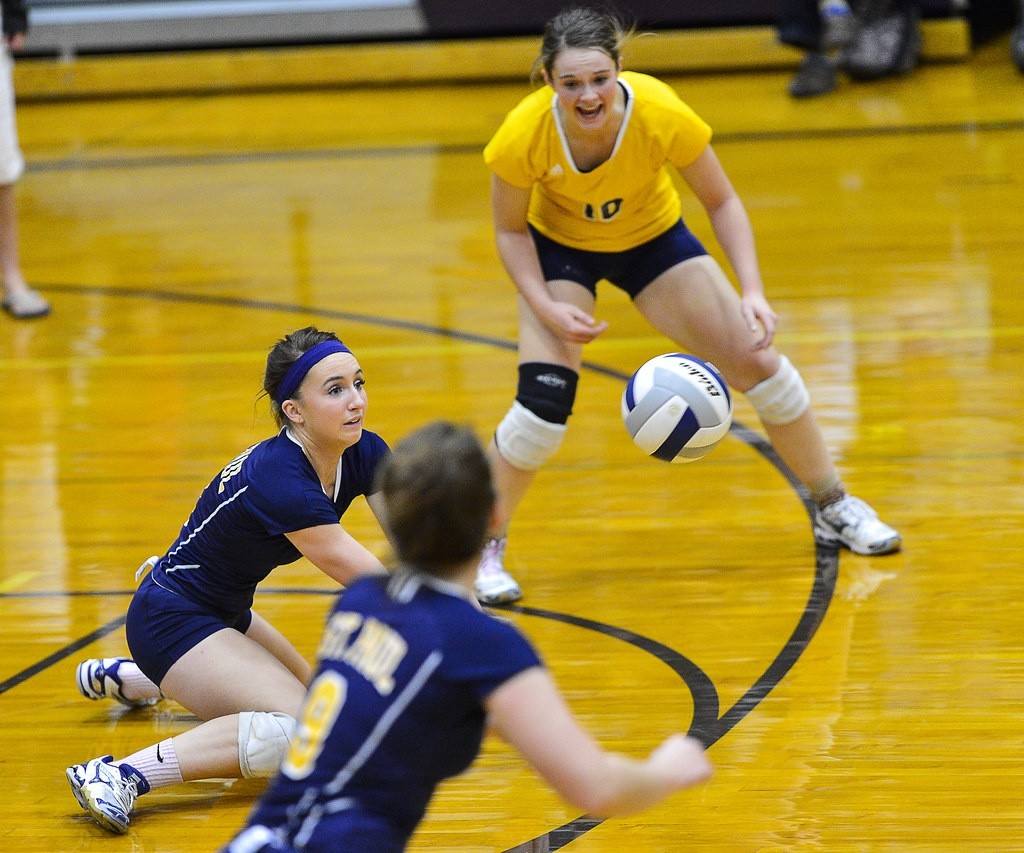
[
  {"x1": 76, "y1": 656, "x2": 161, "y2": 707},
  {"x1": 813, "y1": 490, "x2": 903, "y2": 554},
  {"x1": 473, "y1": 535, "x2": 522, "y2": 604},
  {"x1": 66, "y1": 753, "x2": 150, "y2": 835}
]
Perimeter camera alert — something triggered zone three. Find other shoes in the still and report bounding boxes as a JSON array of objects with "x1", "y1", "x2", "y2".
[
  {"x1": 789, "y1": 57, "x2": 836, "y2": 97},
  {"x1": 0, "y1": 278, "x2": 49, "y2": 318}
]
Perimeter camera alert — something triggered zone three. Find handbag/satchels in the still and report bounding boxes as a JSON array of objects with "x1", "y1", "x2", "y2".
[{"x1": 845, "y1": 2, "x2": 912, "y2": 72}]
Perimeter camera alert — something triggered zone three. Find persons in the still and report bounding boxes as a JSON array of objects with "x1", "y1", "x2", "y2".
[
  {"x1": 65, "y1": 326, "x2": 397, "y2": 835},
  {"x1": 472, "y1": 8, "x2": 901, "y2": 605},
  {"x1": 0, "y1": 0, "x2": 53, "y2": 319},
  {"x1": 772, "y1": 0, "x2": 920, "y2": 100},
  {"x1": 214, "y1": 417, "x2": 712, "y2": 853}
]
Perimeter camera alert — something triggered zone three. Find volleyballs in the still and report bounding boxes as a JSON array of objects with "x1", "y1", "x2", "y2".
[{"x1": 622, "y1": 351, "x2": 734, "y2": 468}]
